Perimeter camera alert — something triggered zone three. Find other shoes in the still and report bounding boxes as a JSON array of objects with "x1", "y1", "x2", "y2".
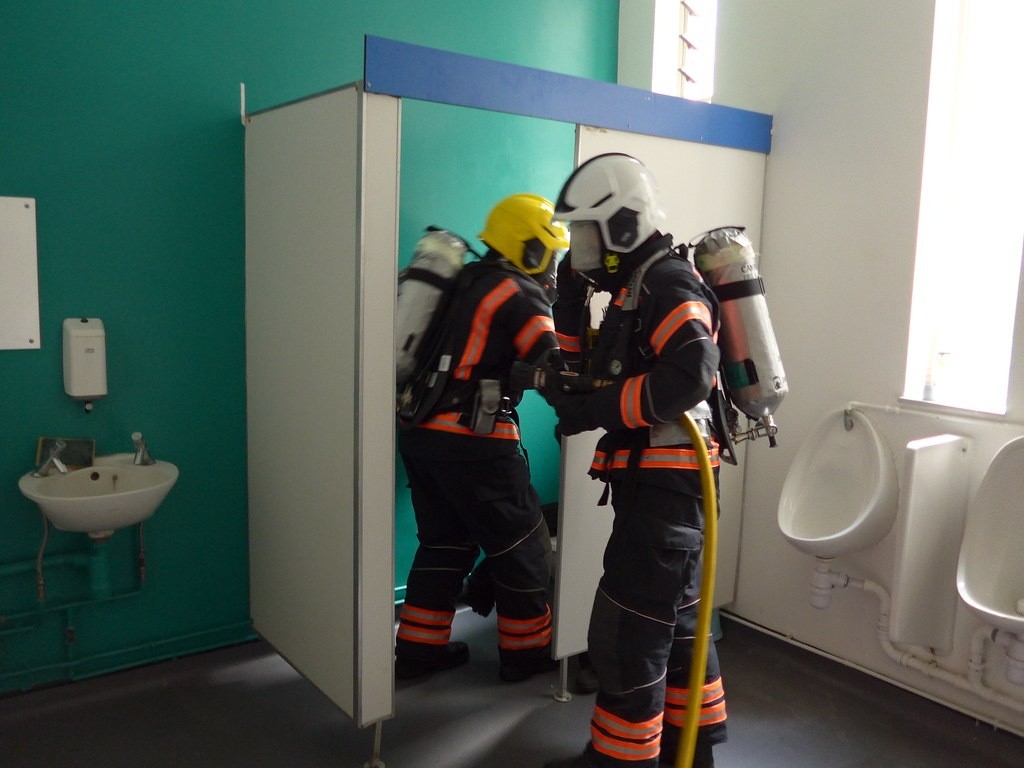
[
  {"x1": 659, "y1": 733, "x2": 716, "y2": 768},
  {"x1": 544, "y1": 741, "x2": 661, "y2": 768},
  {"x1": 395, "y1": 641, "x2": 471, "y2": 682},
  {"x1": 498, "y1": 653, "x2": 560, "y2": 682}
]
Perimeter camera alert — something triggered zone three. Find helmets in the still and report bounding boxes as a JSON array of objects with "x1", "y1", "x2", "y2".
[
  {"x1": 477, "y1": 193, "x2": 570, "y2": 276},
  {"x1": 549, "y1": 152, "x2": 665, "y2": 256}
]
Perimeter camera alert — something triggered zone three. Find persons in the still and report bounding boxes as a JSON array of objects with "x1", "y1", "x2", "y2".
[
  {"x1": 551, "y1": 153, "x2": 729, "y2": 768},
  {"x1": 395, "y1": 194, "x2": 570, "y2": 686}
]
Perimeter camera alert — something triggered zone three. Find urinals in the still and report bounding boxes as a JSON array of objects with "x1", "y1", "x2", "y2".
[
  {"x1": 955, "y1": 436, "x2": 1024, "y2": 635},
  {"x1": 776, "y1": 407, "x2": 901, "y2": 558}
]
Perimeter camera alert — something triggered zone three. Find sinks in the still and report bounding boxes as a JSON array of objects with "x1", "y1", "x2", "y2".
[{"x1": 18, "y1": 452, "x2": 179, "y2": 533}]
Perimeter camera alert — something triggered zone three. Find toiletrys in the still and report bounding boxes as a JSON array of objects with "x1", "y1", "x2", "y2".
[{"x1": 62, "y1": 317, "x2": 108, "y2": 412}]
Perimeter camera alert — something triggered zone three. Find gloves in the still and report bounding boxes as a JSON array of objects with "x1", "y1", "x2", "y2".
[
  {"x1": 544, "y1": 385, "x2": 627, "y2": 439},
  {"x1": 552, "y1": 281, "x2": 592, "y2": 360}
]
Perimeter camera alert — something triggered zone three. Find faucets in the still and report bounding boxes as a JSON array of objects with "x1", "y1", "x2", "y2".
[
  {"x1": 131, "y1": 431, "x2": 157, "y2": 466},
  {"x1": 31, "y1": 438, "x2": 70, "y2": 478}
]
